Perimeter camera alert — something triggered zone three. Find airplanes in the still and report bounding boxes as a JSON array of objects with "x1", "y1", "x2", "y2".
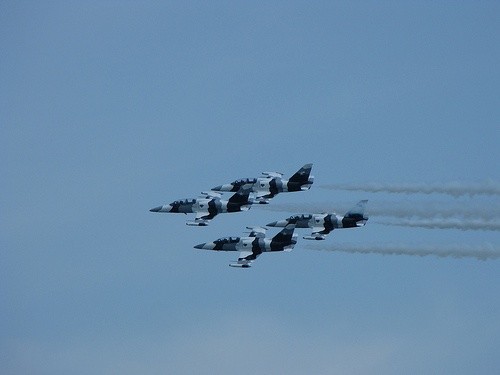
[
  {"x1": 266, "y1": 199, "x2": 369, "y2": 240},
  {"x1": 193, "y1": 224, "x2": 299, "y2": 267},
  {"x1": 212, "y1": 161, "x2": 316, "y2": 203},
  {"x1": 149, "y1": 192, "x2": 258, "y2": 226}
]
